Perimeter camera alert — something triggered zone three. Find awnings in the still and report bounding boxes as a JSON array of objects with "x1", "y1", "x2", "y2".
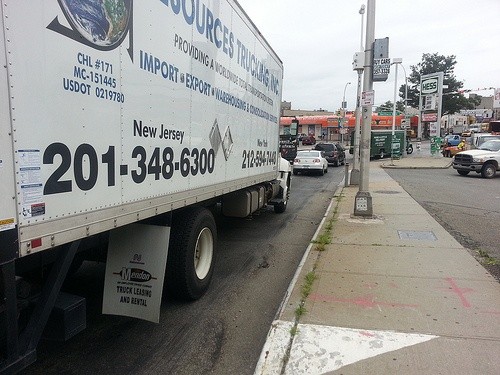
[
  {"x1": 321, "y1": 114, "x2": 420, "y2": 128},
  {"x1": 279, "y1": 115, "x2": 324, "y2": 125}
]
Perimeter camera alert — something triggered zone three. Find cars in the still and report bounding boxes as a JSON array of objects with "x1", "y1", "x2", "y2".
[
  {"x1": 298, "y1": 133, "x2": 316, "y2": 145},
  {"x1": 292, "y1": 149, "x2": 328, "y2": 176},
  {"x1": 440, "y1": 122, "x2": 500, "y2": 179}
]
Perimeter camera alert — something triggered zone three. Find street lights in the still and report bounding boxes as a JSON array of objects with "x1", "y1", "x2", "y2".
[
  {"x1": 390, "y1": 61, "x2": 408, "y2": 158},
  {"x1": 341, "y1": 81, "x2": 351, "y2": 145}
]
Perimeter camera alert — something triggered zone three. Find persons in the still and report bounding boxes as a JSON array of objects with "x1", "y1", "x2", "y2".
[{"x1": 457, "y1": 140, "x2": 465, "y2": 148}]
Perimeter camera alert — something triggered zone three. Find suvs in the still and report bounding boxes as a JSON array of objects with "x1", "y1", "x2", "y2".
[{"x1": 312, "y1": 141, "x2": 347, "y2": 167}]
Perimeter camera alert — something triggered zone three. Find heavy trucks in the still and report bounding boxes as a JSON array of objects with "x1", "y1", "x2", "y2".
[{"x1": 0, "y1": 0, "x2": 300, "y2": 375}]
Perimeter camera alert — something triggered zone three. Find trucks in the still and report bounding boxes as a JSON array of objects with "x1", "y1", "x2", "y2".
[{"x1": 349, "y1": 129, "x2": 413, "y2": 160}]
